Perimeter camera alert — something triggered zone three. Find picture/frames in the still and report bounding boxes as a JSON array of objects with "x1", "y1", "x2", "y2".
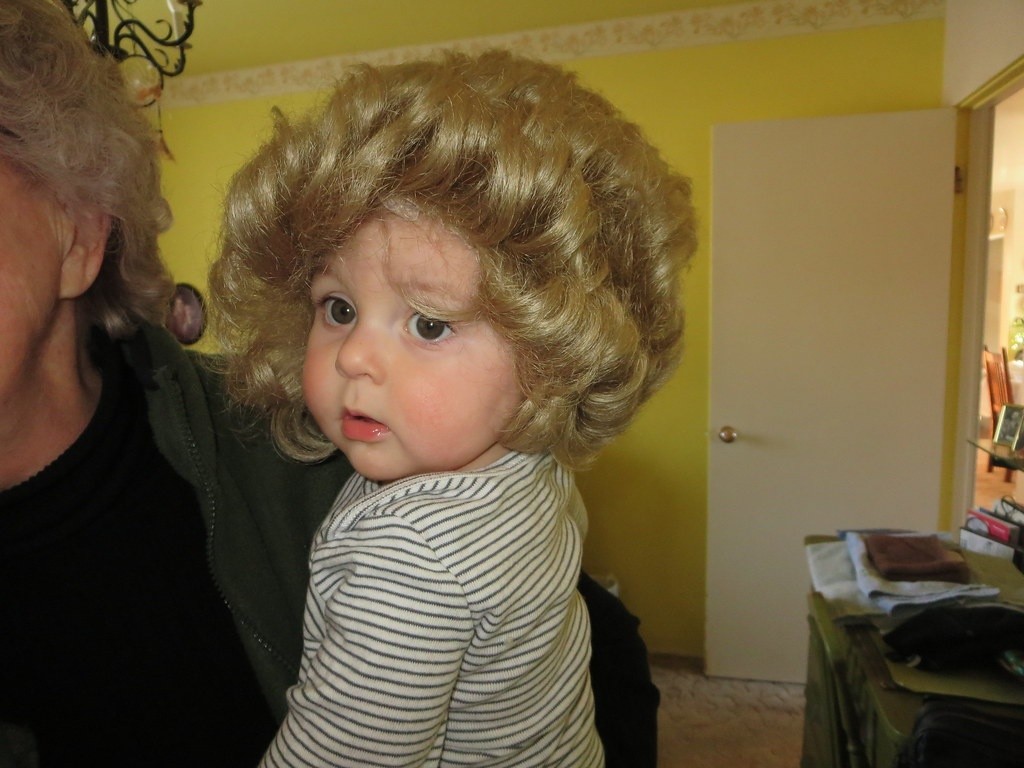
[{"x1": 992, "y1": 402, "x2": 1024, "y2": 453}]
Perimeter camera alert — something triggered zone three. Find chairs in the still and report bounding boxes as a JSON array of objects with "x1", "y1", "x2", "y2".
[{"x1": 983, "y1": 345, "x2": 1016, "y2": 483}]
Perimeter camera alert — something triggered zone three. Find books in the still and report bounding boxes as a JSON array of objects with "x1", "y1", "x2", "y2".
[{"x1": 959, "y1": 498, "x2": 1024, "y2": 571}]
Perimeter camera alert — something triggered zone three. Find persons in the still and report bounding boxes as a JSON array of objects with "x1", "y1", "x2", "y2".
[
  {"x1": 203, "y1": 48, "x2": 699, "y2": 768},
  {"x1": 0, "y1": 1, "x2": 659, "y2": 768}
]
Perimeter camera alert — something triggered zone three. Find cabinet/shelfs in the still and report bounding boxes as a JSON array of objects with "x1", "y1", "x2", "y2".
[{"x1": 796, "y1": 532, "x2": 1024, "y2": 768}]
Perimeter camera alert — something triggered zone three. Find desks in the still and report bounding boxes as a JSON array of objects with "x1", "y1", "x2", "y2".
[{"x1": 980, "y1": 364, "x2": 1024, "y2": 419}]
